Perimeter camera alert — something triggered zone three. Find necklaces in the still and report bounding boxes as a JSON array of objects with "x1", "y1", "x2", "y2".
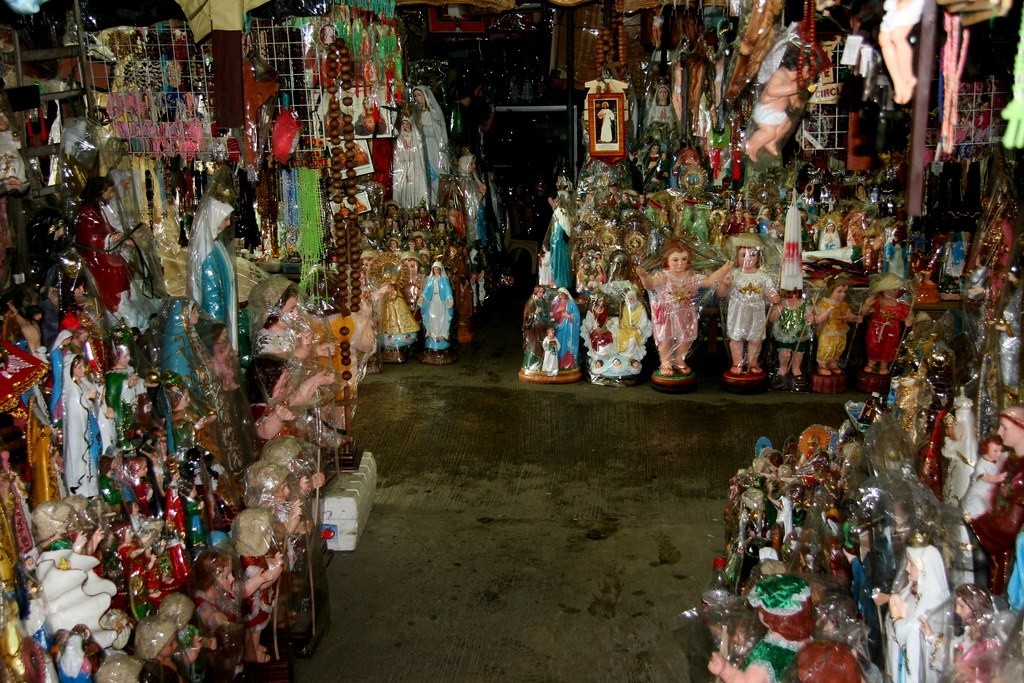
[
  {"x1": 326, "y1": 37, "x2": 361, "y2": 443},
  {"x1": 596, "y1": 0, "x2": 627, "y2": 98},
  {"x1": 798, "y1": 0, "x2": 816, "y2": 89}
]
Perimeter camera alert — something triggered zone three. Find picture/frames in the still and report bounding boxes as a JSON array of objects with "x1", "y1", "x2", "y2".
[
  {"x1": 588, "y1": 92, "x2": 625, "y2": 156},
  {"x1": 427, "y1": 6, "x2": 486, "y2": 33}
]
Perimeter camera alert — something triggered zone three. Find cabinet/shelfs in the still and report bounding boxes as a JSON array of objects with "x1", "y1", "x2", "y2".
[{"x1": 490, "y1": 104, "x2": 579, "y2": 257}]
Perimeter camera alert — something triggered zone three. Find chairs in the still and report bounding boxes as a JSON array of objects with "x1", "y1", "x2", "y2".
[{"x1": 502, "y1": 208, "x2": 539, "y2": 274}]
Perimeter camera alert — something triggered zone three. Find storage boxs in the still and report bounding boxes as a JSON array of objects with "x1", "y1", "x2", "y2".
[{"x1": 309, "y1": 452, "x2": 378, "y2": 552}]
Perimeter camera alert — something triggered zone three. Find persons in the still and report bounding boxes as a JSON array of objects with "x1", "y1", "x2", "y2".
[
  {"x1": 744, "y1": 46, "x2": 805, "y2": 162},
  {"x1": 188, "y1": 196, "x2": 238, "y2": 354},
  {"x1": 597, "y1": 102, "x2": 615, "y2": 144},
  {"x1": 542, "y1": 327, "x2": 561, "y2": 376},
  {"x1": 356, "y1": 98, "x2": 387, "y2": 136},
  {"x1": 393, "y1": 116, "x2": 429, "y2": 210},
  {"x1": 814, "y1": 276, "x2": 864, "y2": 376},
  {"x1": 0, "y1": 94, "x2": 509, "y2": 683},
  {"x1": 417, "y1": 261, "x2": 454, "y2": 340},
  {"x1": 635, "y1": 238, "x2": 735, "y2": 378},
  {"x1": 517, "y1": 0, "x2": 1024, "y2": 683},
  {"x1": 322, "y1": 97, "x2": 351, "y2": 136},
  {"x1": 818, "y1": 219, "x2": 840, "y2": 251},
  {"x1": 718, "y1": 233, "x2": 782, "y2": 375},
  {"x1": 306, "y1": 24, "x2": 338, "y2": 85},
  {"x1": 861, "y1": 272, "x2": 913, "y2": 375},
  {"x1": 61, "y1": 353, "x2": 99, "y2": 498}
]
